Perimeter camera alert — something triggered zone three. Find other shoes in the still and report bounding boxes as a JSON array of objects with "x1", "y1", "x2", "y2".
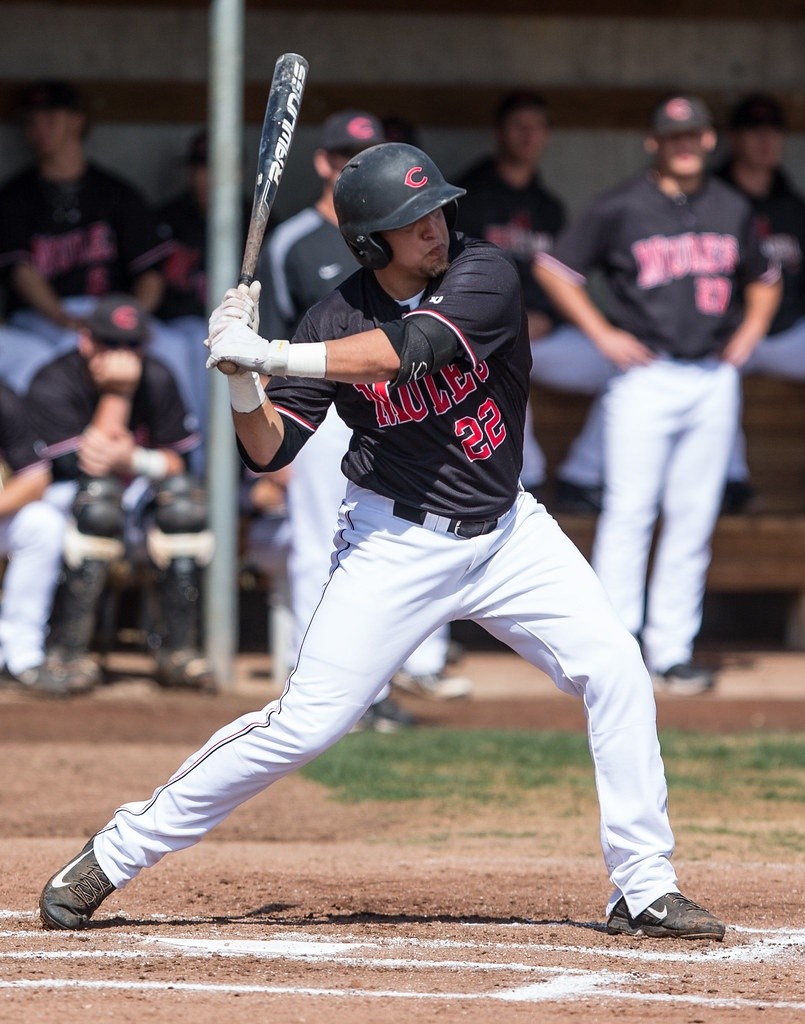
[
  {"x1": 559, "y1": 471, "x2": 605, "y2": 512},
  {"x1": 530, "y1": 476, "x2": 556, "y2": 512},
  {"x1": 157, "y1": 648, "x2": 212, "y2": 686},
  {"x1": 17, "y1": 649, "x2": 99, "y2": 691}
]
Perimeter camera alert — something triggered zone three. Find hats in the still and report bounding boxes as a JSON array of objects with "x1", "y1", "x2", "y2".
[
  {"x1": 651, "y1": 94, "x2": 712, "y2": 139},
  {"x1": 87, "y1": 296, "x2": 149, "y2": 342},
  {"x1": 318, "y1": 112, "x2": 385, "y2": 150}
]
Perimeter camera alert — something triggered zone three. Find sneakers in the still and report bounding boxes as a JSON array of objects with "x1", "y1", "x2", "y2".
[
  {"x1": 605, "y1": 893, "x2": 725, "y2": 941},
  {"x1": 657, "y1": 664, "x2": 715, "y2": 695},
  {"x1": 38, "y1": 829, "x2": 117, "y2": 929}
]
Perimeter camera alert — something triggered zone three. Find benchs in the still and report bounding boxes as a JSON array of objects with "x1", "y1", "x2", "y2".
[{"x1": 1, "y1": 365, "x2": 805, "y2": 652}]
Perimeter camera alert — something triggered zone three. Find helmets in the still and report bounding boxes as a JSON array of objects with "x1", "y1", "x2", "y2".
[{"x1": 333, "y1": 142, "x2": 466, "y2": 271}]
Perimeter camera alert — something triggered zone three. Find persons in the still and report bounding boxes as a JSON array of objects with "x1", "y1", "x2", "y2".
[
  {"x1": 135, "y1": 131, "x2": 283, "y2": 511},
  {"x1": 531, "y1": 97, "x2": 783, "y2": 695},
  {"x1": 443, "y1": 84, "x2": 623, "y2": 510},
  {"x1": 251, "y1": 108, "x2": 426, "y2": 738},
  {"x1": 0, "y1": 292, "x2": 209, "y2": 694},
  {"x1": 706, "y1": 89, "x2": 805, "y2": 510},
  {"x1": 40, "y1": 139, "x2": 728, "y2": 941},
  {"x1": 0, "y1": 84, "x2": 207, "y2": 480}
]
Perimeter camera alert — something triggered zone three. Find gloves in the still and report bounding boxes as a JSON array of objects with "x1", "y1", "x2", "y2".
[
  {"x1": 207, "y1": 280, "x2": 266, "y2": 413},
  {"x1": 205, "y1": 320, "x2": 326, "y2": 379}
]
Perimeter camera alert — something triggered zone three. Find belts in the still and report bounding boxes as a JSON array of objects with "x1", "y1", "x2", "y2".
[{"x1": 393, "y1": 502, "x2": 499, "y2": 539}]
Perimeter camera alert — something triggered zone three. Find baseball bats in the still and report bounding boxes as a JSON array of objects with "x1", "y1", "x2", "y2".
[{"x1": 217, "y1": 53, "x2": 309, "y2": 376}]
